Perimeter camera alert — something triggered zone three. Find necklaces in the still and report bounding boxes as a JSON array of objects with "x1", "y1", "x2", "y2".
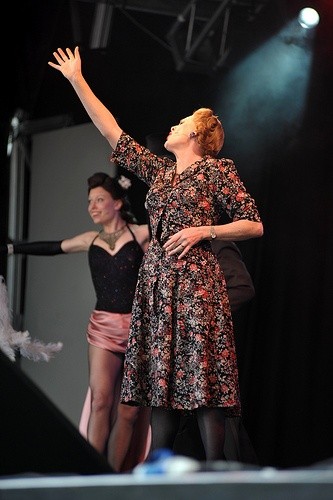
[{"x1": 98, "y1": 224, "x2": 128, "y2": 250}]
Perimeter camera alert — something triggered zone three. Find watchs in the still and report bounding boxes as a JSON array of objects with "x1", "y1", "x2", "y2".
[{"x1": 209, "y1": 225, "x2": 217, "y2": 240}]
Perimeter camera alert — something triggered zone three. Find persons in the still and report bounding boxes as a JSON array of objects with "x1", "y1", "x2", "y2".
[
  {"x1": 206, "y1": 240, "x2": 255, "y2": 472},
  {"x1": 6, "y1": 173, "x2": 152, "y2": 472},
  {"x1": 47, "y1": 46, "x2": 263, "y2": 472}
]
